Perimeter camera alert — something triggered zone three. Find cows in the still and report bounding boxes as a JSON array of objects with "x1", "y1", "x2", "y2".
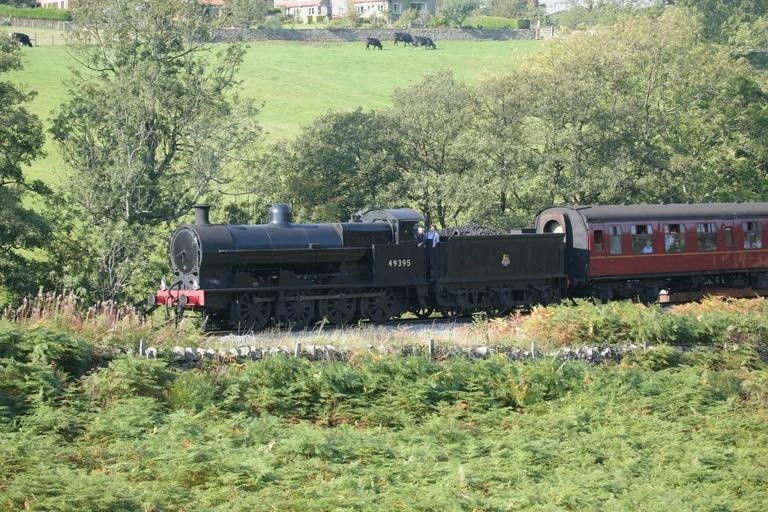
[
  {"x1": 11, "y1": 32, "x2": 32, "y2": 48},
  {"x1": 365, "y1": 38, "x2": 382, "y2": 51},
  {"x1": 414, "y1": 36, "x2": 436, "y2": 50},
  {"x1": 393, "y1": 33, "x2": 414, "y2": 47}
]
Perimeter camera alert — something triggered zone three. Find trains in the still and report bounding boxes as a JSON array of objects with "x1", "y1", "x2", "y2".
[{"x1": 148, "y1": 201, "x2": 767, "y2": 335}]
